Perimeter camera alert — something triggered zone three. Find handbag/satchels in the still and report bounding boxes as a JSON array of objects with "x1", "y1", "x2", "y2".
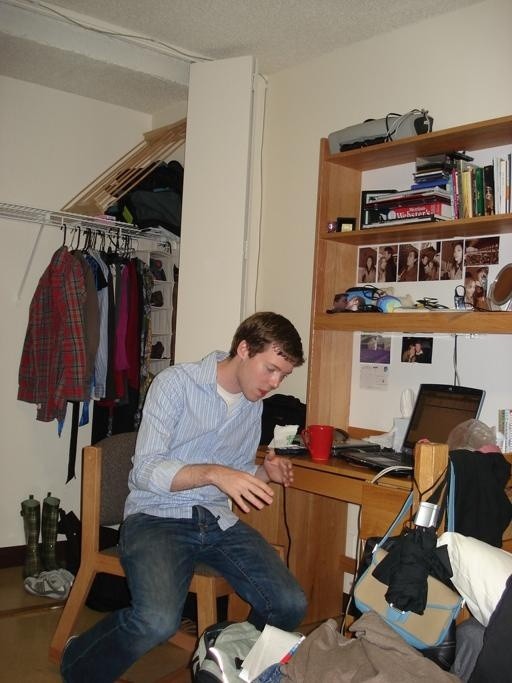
[{"x1": 353, "y1": 463, "x2": 464, "y2": 650}]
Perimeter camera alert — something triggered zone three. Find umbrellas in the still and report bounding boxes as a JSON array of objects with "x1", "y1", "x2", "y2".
[{"x1": 371, "y1": 500, "x2": 440, "y2": 614}]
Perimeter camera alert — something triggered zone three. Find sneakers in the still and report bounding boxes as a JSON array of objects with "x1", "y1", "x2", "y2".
[
  {"x1": 23, "y1": 573, "x2": 70, "y2": 600},
  {"x1": 39, "y1": 567, "x2": 75, "y2": 587}
]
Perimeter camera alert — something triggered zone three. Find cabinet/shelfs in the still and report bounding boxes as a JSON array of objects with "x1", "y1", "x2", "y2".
[{"x1": 226, "y1": 113, "x2": 510, "y2": 626}]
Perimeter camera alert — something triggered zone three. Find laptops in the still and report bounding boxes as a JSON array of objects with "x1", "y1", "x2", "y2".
[{"x1": 340, "y1": 382, "x2": 487, "y2": 478}]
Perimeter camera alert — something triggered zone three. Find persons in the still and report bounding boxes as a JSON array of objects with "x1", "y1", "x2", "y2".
[
  {"x1": 61, "y1": 309, "x2": 308, "y2": 681},
  {"x1": 441, "y1": 243, "x2": 462, "y2": 280},
  {"x1": 403, "y1": 342, "x2": 416, "y2": 360},
  {"x1": 383, "y1": 245, "x2": 397, "y2": 282},
  {"x1": 414, "y1": 343, "x2": 426, "y2": 362},
  {"x1": 420, "y1": 254, "x2": 429, "y2": 281},
  {"x1": 378, "y1": 257, "x2": 387, "y2": 282},
  {"x1": 342, "y1": 296, "x2": 366, "y2": 312},
  {"x1": 399, "y1": 248, "x2": 417, "y2": 281},
  {"x1": 464, "y1": 272, "x2": 477, "y2": 310},
  {"x1": 361, "y1": 255, "x2": 376, "y2": 282},
  {"x1": 325, "y1": 291, "x2": 357, "y2": 313},
  {"x1": 475, "y1": 266, "x2": 490, "y2": 311}
]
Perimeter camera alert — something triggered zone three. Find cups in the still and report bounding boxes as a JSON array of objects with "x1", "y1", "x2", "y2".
[{"x1": 301, "y1": 425, "x2": 334, "y2": 461}]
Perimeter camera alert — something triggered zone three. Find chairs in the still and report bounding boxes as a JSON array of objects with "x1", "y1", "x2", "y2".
[{"x1": 45, "y1": 427, "x2": 235, "y2": 664}]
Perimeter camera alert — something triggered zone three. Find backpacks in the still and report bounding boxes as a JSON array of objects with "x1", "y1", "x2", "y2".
[{"x1": 189, "y1": 620, "x2": 262, "y2": 683}]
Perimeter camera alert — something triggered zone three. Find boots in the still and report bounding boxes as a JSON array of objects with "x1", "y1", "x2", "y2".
[
  {"x1": 42, "y1": 491, "x2": 60, "y2": 570},
  {"x1": 63, "y1": 538, "x2": 78, "y2": 570},
  {"x1": 20, "y1": 494, "x2": 40, "y2": 579},
  {"x1": 59, "y1": 511, "x2": 81, "y2": 565}
]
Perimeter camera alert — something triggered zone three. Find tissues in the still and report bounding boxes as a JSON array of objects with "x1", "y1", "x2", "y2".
[{"x1": 391, "y1": 388, "x2": 416, "y2": 456}]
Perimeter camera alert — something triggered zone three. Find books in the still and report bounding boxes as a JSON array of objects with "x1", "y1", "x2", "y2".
[{"x1": 359, "y1": 154, "x2": 511, "y2": 229}]
